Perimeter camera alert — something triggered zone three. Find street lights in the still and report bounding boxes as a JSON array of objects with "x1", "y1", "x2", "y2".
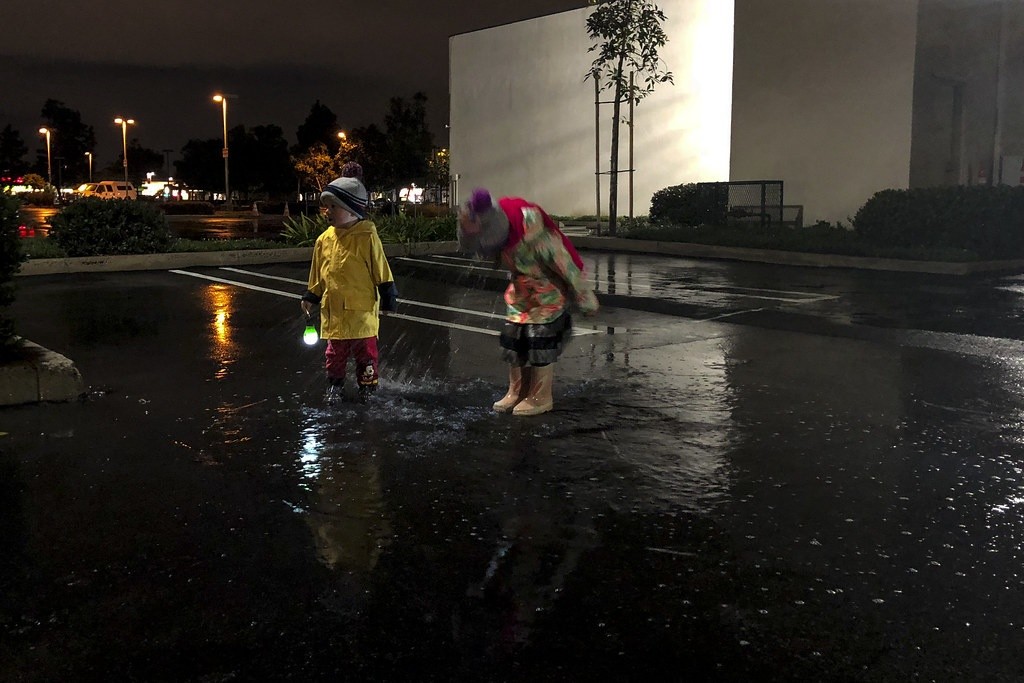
[
  {"x1": 337, "y1": 131, "x2": 350, "y2": 162},
  {"x1": 114, "y1": 118, "x2": 136, "y2": 200},
  {"x1": 84, "y1": 152, "x2": 92, "y2": 183},
  {"x1": 212, "y1": 94, "x2": 229, "y2": 199},
  {"x1": 38, "y1": 127, "x2": 51, "y2": 185}
]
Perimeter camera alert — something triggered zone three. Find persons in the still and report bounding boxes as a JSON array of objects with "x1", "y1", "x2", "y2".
[
  {"x1": 310, "y1": 448, "x2": 394, "y2": 608},
  {"x1": 302, "y1": 161, "x2": 399, "y2": 404},
  {"x1": 458, "y1": 186, "x2": 600, "y2": 417}
]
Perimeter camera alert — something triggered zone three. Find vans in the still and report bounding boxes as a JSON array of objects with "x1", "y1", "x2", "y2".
[{"x1": 73, "y1": 179, "x2": 136, "y2": 201}]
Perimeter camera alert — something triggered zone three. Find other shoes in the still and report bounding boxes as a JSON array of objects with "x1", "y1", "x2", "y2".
[
  {"x1": 355, "y1": 359, "x2": 379, "y2": 386},
  {"x1": 321, "y1": 385, "x2": 348, "y2": 406}
]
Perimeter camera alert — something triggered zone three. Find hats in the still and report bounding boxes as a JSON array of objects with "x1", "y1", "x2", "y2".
[
  {"x1": 455, "y1": 186, "x2": 509, "y2": 256},
  {"x1": 319, "y1": 161, "x2": 368, "y2": 219}
]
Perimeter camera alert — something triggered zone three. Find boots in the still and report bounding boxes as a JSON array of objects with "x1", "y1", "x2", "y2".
[
  {"x1": 492, "y1": 366, "x2": 530, "y2": 414},
  {"x1": 512, "y1": 363, "x2": 553, "y2": 416}
]
